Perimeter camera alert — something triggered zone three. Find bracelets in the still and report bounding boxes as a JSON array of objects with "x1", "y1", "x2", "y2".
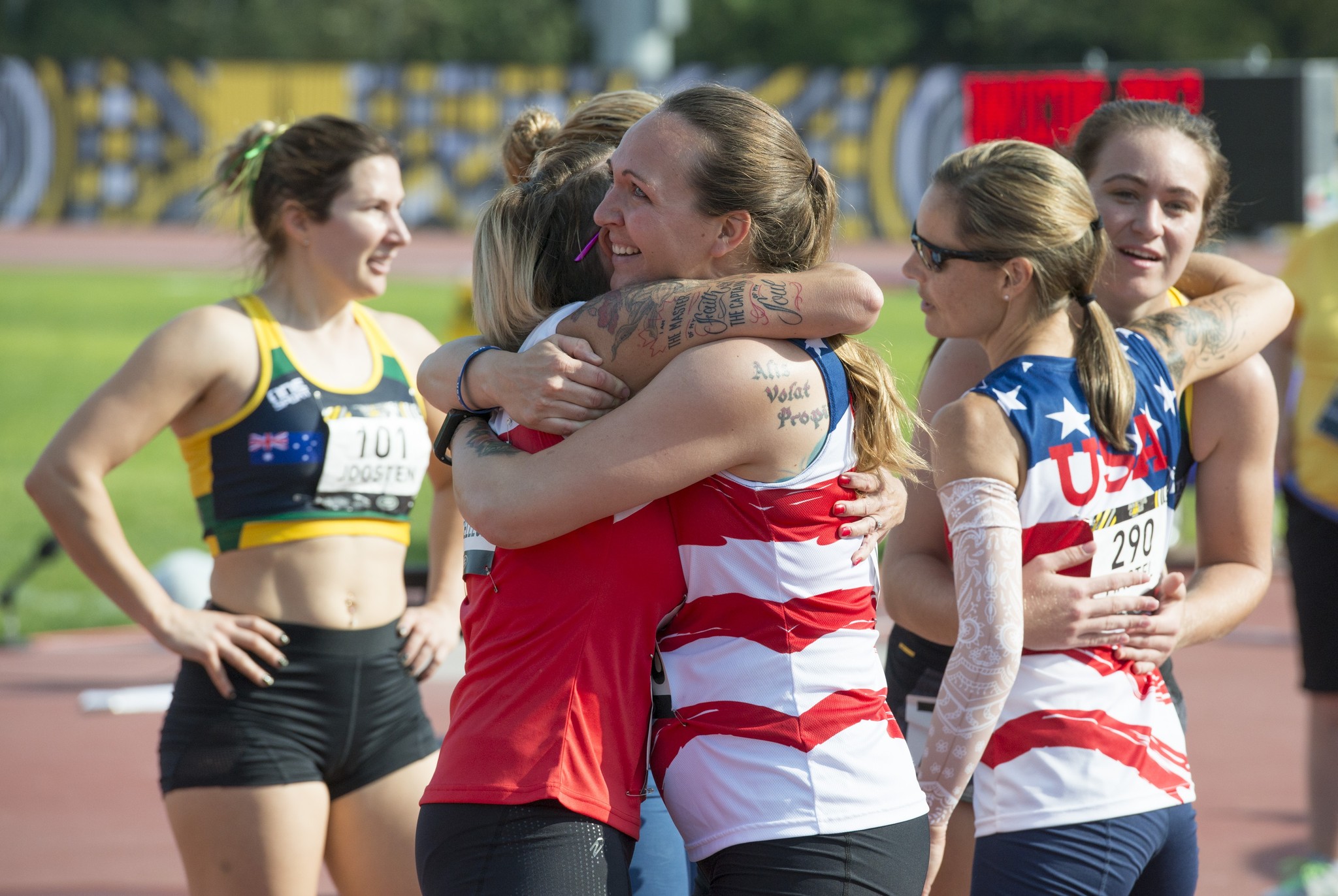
[{"x1": 455, "y1": 342, "x2": 498, "y2": 415}]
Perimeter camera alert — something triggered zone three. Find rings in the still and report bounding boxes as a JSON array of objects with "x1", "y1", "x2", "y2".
[{"x1": 870, "y1": 514, "x2": 883, "y2": 534}]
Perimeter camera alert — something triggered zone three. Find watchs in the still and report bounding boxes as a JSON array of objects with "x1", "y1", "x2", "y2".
[{"x1": 430, "y1": 407, "x2": 469, "y2": 466}]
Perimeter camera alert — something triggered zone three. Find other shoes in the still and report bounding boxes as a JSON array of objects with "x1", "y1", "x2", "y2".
[{"x1": 1266, "y1": 853, "x2": 1338, "y2": 896}]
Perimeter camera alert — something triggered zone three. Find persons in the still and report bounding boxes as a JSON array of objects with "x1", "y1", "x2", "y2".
[
  {"x1": 22, "y1": 109, "x2": 468, "y2": 896},
  {"x1": 414, "y1": 84, "x2": 933, "y2": 896},
  {"x1": 878, "y1": 95, "x2": 1294, "y2": 896},
  {"x1": 1259, "y1": 211, "x2": 1338, "y2": 867},
  {"x1": 503, "y1": 93, "x2": 691, "y2": 896},
  {"x1": 412, "y1": 82, "x2": 905, "y2": 896}
]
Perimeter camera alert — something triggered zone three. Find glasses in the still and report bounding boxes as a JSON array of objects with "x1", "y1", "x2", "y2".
[{"x1": 911, "y1": 219, "x2": 1019, "y2": 273}]
